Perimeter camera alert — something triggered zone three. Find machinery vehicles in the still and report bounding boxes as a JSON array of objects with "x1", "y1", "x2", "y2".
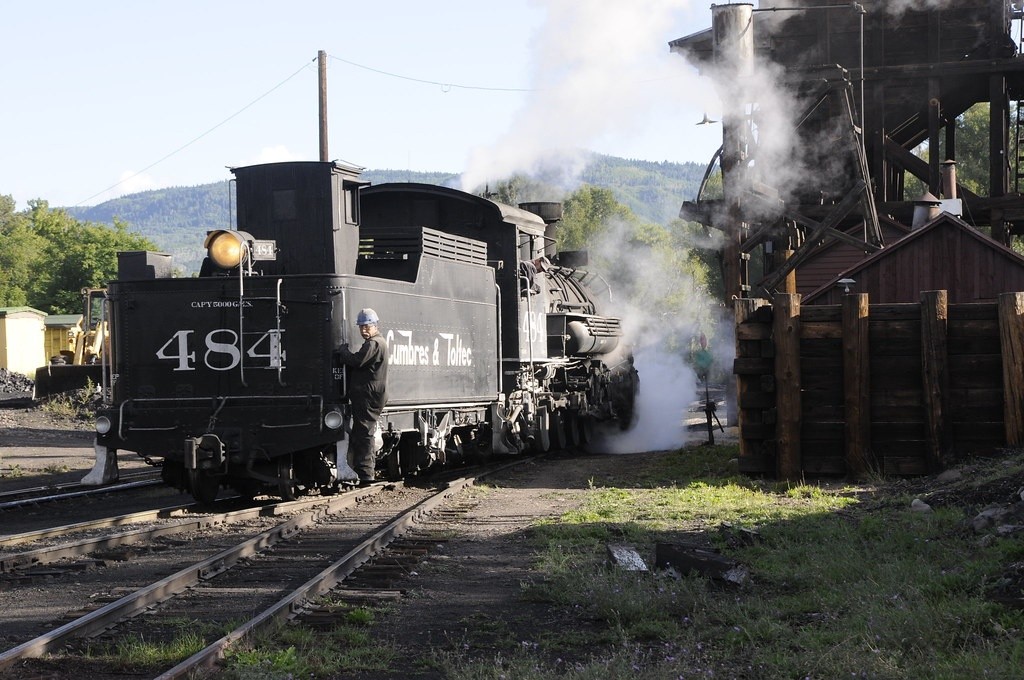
[{"x1": 30, "y1": 286, "x2": 111, "y2": 394}]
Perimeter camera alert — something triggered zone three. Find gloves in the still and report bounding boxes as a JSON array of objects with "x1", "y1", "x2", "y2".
[{"x1": 336, "y1": 343, "x2": 351, "y2": 357}]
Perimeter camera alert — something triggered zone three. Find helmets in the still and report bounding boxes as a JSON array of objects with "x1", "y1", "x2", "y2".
[{"x1": 357, "y1": 308, "x2": 379, "y2": 325}]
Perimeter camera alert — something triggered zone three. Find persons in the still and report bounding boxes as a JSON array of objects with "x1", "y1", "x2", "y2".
[{"x1": 334, "y1": 308, "x2": 389, "y2": 482}]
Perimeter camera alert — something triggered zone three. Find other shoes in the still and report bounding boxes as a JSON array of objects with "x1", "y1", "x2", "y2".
[{"x1": 344, "y1": 479, "x2": 374, "y2": 487}]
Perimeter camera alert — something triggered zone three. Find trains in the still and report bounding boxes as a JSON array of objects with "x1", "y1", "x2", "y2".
[{"x1": 96, "y1": 158, "x2": 639, "y2": 508}]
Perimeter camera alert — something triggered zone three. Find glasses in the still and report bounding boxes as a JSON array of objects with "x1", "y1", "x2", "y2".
[{"x1": 359, "y1": 325, "x2": 375, "y2": 330}]
